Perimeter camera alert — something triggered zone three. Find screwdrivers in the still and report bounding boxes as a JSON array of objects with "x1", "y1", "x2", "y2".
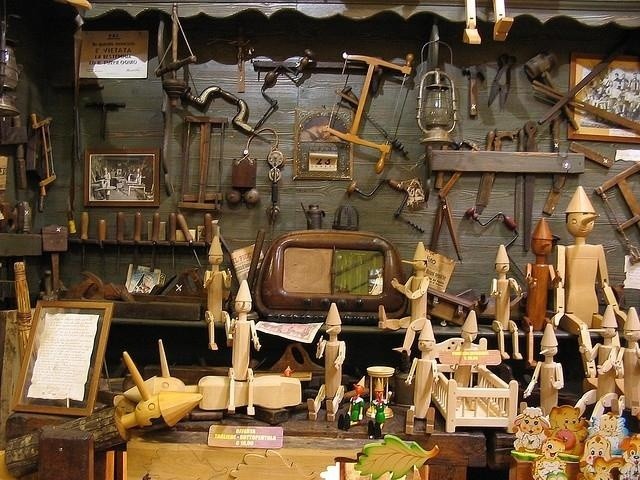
[{"x1": 81, "y1": 212, "x2": 213, "y2": 273}]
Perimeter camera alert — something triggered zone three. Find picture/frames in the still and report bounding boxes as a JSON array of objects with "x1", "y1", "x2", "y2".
[
  {"x1": 10, "y1": 299, "x2": 114, "y2": 417},
  {"x1": 566, "y1": 51, "x2": 640, "y2": 144},
  {"x1": 83, "y1": 148, "x2": 161, "y2": 209}
]
